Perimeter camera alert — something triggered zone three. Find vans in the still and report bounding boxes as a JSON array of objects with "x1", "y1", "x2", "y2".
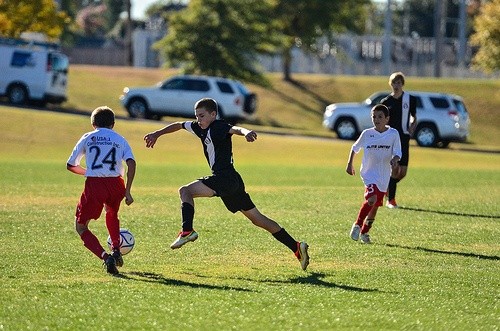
[{"x1": 0, "y1": 34, "x2": 70, "y2": 107}]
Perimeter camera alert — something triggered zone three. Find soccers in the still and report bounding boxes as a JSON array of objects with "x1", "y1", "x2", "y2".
[{"x1": 107, "y1": 229, "x2": 136, "y2": 256}]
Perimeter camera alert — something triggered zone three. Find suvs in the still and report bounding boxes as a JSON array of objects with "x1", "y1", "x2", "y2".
[
  {"x1": 119, "y1": 72, "x2": 259, "y2": 126},
  {"x1": 323, "y1": 87, "x2": 472, "y2": 151}
]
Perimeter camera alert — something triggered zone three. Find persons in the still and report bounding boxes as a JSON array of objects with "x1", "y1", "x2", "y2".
[
  {"x1": 379, "y1": 73, "x2": 420, "y2": 210},
  {"x1": 66, "y1": 106, "x2": 137, "y2": 274},
  {"x1": 144, "y1": 98, "x2": 310, "y2": 272},
  {"x1": 346, "y1": 104, "x2": 402, "y2": 245}
]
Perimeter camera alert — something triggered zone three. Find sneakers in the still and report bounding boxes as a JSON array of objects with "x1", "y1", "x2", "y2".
[
  {"x1": 386, "y1": 200, "x2": 397, "y2": 208},
  {"x1": 111, "y1": 249, "x2": 123, "y2": 267},
  {"x1": 170, "y1": 230, "x2": 198, "y2": 249},
  {"x1": 102, "y1": 254, "x2": 119, "y2": 275},
  {"x1": 294, "y1": 242, "x2": 310, "y2": 271},
  {"x1": 360, "y1": 233, "x2": 370, "y2": 244},
  {"x1": 350, "y1": 224, "x2": 360, "y2": 241}
]
